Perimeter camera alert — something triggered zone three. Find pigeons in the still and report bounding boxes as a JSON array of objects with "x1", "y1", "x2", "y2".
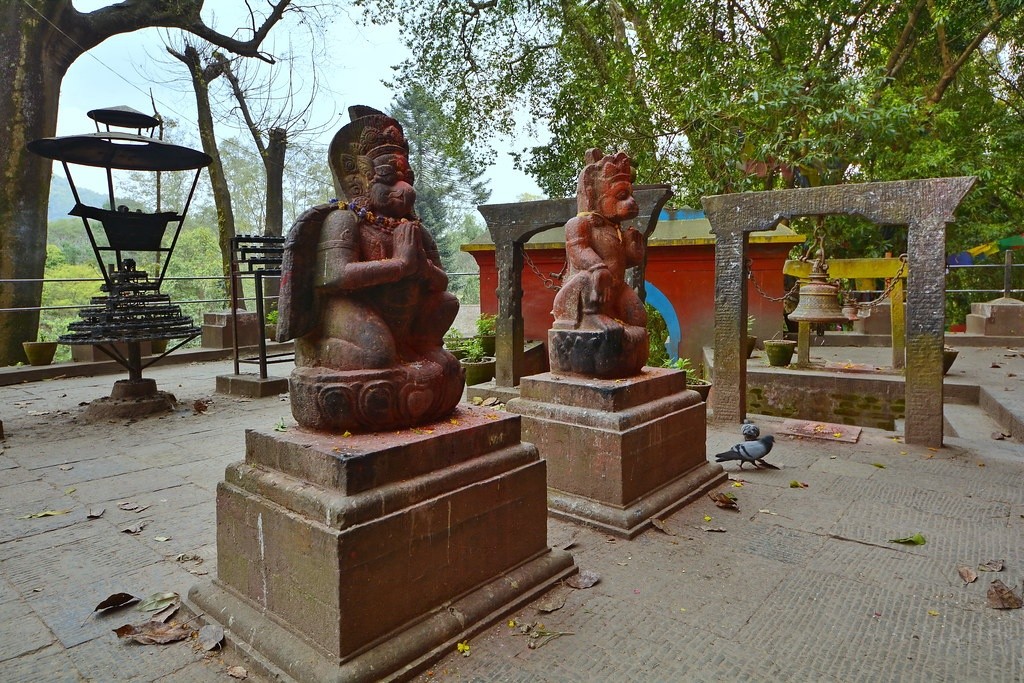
[
  {"x1": 741, "y1": 423, "x2": 760, "y2": 442},
  {"x1": 715, "y1": 435, "x2": 776, "y2": 470}
]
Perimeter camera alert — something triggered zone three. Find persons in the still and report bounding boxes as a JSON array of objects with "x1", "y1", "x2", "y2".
[
  {"x1": 551, "y1": 149, "x2": 651, "y2": 339},
  {"x1": 281, "y1": 105, "x2": 469, "y2": 429}
]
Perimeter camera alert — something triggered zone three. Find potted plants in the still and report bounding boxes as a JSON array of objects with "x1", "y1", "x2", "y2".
[
  {"x1": 661, "y1": 357, "x2": 713, "y2": 403},
  {"x1": 22, "y1": 332, "x2": 59, "y2": 366},
  {"x1": 265, "y1": 301, "x2": 280, "y2": 342},
  {"x1": 746, "y1": 314, "x2": 757, "y2": 359},
  {"x1": 443, "y1": 312, "x2": 499, "y2": 386}
]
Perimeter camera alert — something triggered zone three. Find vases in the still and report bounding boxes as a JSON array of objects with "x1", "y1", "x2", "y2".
[
  {"x1": 762, "y1": 332, "x2": 798, "y2": 366},
  {"x1": 944, "y1": 347, "x2": 959, "y2": 375}
]
